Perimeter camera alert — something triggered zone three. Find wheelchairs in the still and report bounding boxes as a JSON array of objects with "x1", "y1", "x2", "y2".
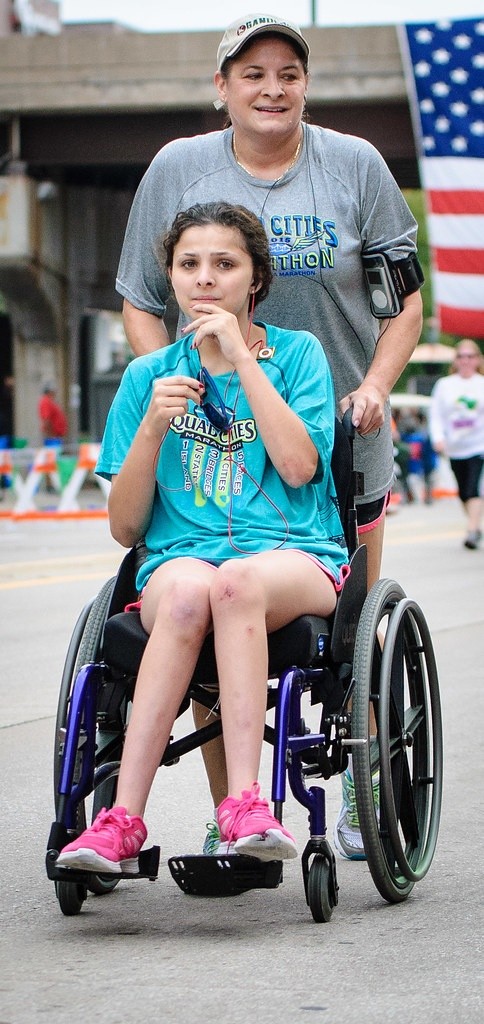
[{"x1": 43, "y1": 404, "x2": 444, "y2": 923}]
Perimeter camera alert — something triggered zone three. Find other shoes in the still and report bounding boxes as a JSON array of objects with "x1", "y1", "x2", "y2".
[{"x1": 465, "y1": 527, "x2": 482, "y2": 549}]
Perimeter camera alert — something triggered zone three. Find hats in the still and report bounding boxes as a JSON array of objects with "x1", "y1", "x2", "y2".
[{"x1": 213, "y1": 12, "x2": 310, "y2": 110}]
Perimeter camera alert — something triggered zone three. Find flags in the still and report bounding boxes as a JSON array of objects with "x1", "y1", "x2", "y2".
[{"x1": 399, "y1": 21, "x2": 484, "y2": 338}]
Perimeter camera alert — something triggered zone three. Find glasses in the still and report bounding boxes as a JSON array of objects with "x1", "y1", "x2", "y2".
[{"x1": 197, "y1": 367, "x2": 228, "y2": 432}]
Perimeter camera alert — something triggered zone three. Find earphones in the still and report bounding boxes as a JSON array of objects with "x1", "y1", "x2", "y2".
[{"x1": 251, "y1": 278, "x2": 260, "y2": 291}]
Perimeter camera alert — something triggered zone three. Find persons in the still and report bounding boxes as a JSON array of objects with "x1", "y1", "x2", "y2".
[
  {"x1": 57, "y1": 202, "x2": 351, "y2": 876},
  {"x1": 40, "y1": 338, "x2": 484, "y2": 553},
  {"x1": 117, "y1": 13, "x2": 426, "y2": 854}
]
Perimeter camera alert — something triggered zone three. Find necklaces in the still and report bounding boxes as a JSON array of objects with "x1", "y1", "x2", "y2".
[{"x1": 231, "y1": 124, "x2": 304, "y2": 172}]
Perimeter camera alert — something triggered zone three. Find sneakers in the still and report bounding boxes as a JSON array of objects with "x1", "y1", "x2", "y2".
[
  {"x1": 54, "y1": 807, "x2": 147, "y2": 873},
  {"x1": 202, "y1": 819, "x2": 238, "y2": 854},
  {"x1": 217, "y1": 781, "x2": 297, "y2": 861},
  {"x1": 332, "y1": 766, "x2": 380, "y2": 860}
]
